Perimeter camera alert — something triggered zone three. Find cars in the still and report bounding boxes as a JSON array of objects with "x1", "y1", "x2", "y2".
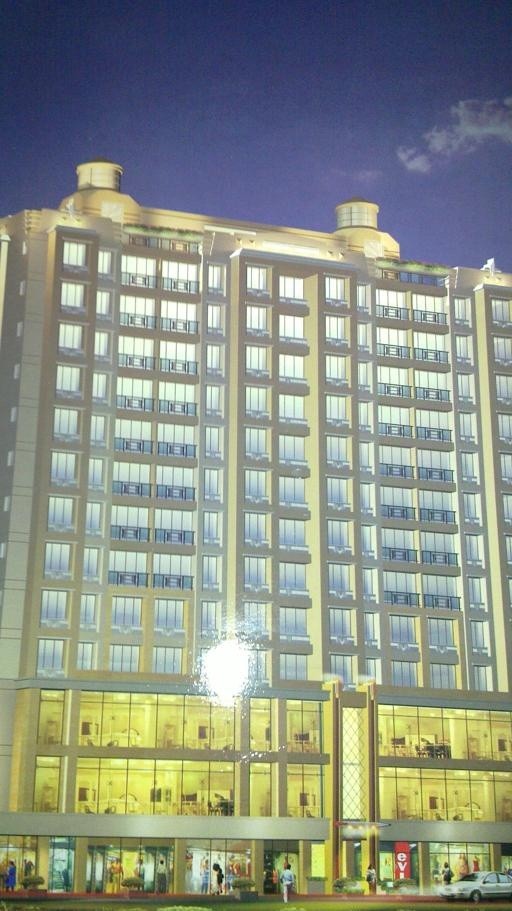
[{"x1": 433, "y1": 866, "x2": 512, "y2": 903}]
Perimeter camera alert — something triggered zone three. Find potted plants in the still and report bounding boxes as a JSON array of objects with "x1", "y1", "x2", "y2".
[
  {"x1": 232, "y1": 880, "x2": 259, "y2": 902},
  {"x1": 17, "y1": 874, "x2": 47, "y2": 894},
  {"x1": 306, "y1": 875, "x2": 325, "y2": 895},
  {"x1": 122, "y1": 876, "x2": 149, "y2": 899},
  {"x1": 394, "y1": 878, "x2": 420, "y2": 896},
  {"x1": 348, "y1": 887, "x2": 365, "y2": 897}
]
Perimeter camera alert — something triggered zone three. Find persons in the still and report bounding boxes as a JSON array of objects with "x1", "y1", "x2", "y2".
[
  {"x1": 201, "y1": 867, "x2": 209, "y2": 894},
  {"x1": 134, "y1": 860, "x2": 144, "y2": 891},
  {"x1": 442, "y1": 862, "x2": 454, "y2": 884},
  {"x1": 110, "y1": 858, "x2": 124, "y2": 893},
  {"x1": 280, "y1": 864, "x2": 295, "y2": 903},
  {"x1": 156, "y1": 860, "x2": 166, "y2": 893},
  {"x1": 457, "y1": 851, "x2": 479, "y2": 876},
  {"x1": 217, "y1": 868, "x2": 224, "y2": 894},
  {"x1": 366, "y1": 864, "x2": 376, "y2": 895},
  {"x1": 6, "y1": 861, "x2": 16, "y2": 892}
]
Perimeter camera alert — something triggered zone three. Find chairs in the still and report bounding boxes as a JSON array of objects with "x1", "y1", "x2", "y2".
[{"x1": 416, "y1": 745, "x2": 430, "y2": 758}]
